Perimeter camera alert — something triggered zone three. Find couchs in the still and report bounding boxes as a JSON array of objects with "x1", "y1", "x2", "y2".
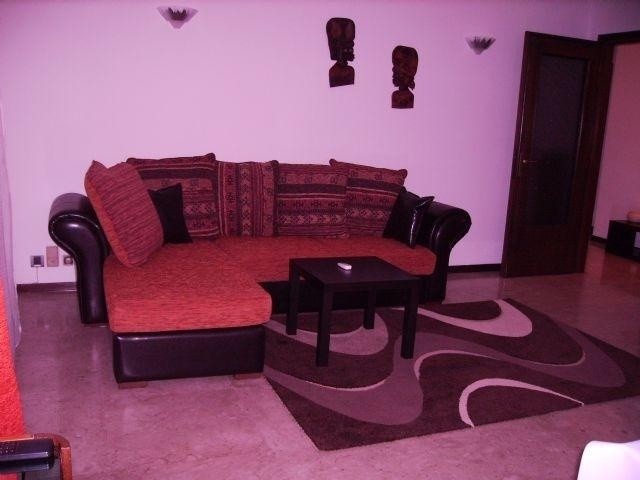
[{"x1": 46, "y1": 153, "x2": 477, "y2": 390}]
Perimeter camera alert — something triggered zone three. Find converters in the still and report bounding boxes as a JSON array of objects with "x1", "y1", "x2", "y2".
[{"x1": 34, "y1": 254, "x2": 41, "y2": 265}]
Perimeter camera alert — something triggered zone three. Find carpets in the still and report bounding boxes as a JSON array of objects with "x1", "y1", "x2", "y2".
[{"x1": 254, "y1": 296, "x2": 639, "y2": 454}]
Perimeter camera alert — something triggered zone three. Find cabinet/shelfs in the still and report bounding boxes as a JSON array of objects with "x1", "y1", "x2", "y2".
[{"x1": 603, "y1": 217, "x2": 640, "y2": 261}]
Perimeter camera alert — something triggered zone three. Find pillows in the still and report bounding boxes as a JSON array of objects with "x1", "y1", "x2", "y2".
[
  {"x1": 80, "y1": 157, "x2": 166, "y2": 266},
  {"x1": 383, "y1": 185, "x2": 435, "y2": 251},
  {"x1": 145, "y1": 183, "x2": 193, "y2": 243},
  {"x1": 275, "y1": 159, "x2": 352, "y2": 242},
  {"x1": 123, "y1": 153, "x2": 222, "y2": 243},
  {"x1": 216, "y1": 157, "x2": 281, "y2": 238},
  {"x1": 328, "y1": 157, "x2": 407, "y2": 238}
]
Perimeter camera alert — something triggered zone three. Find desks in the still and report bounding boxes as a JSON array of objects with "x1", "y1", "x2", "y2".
[{"x1": 286, "y1": 255, "x2": 427, "y2": 370}]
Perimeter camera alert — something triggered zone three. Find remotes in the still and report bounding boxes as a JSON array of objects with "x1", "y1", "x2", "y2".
[
  {"x1": 0, "y1": 439, "x2": 55, "y2": 462},
  {"x1": 337, "y1": 261, "x2": 352, "y2": 271}
]
